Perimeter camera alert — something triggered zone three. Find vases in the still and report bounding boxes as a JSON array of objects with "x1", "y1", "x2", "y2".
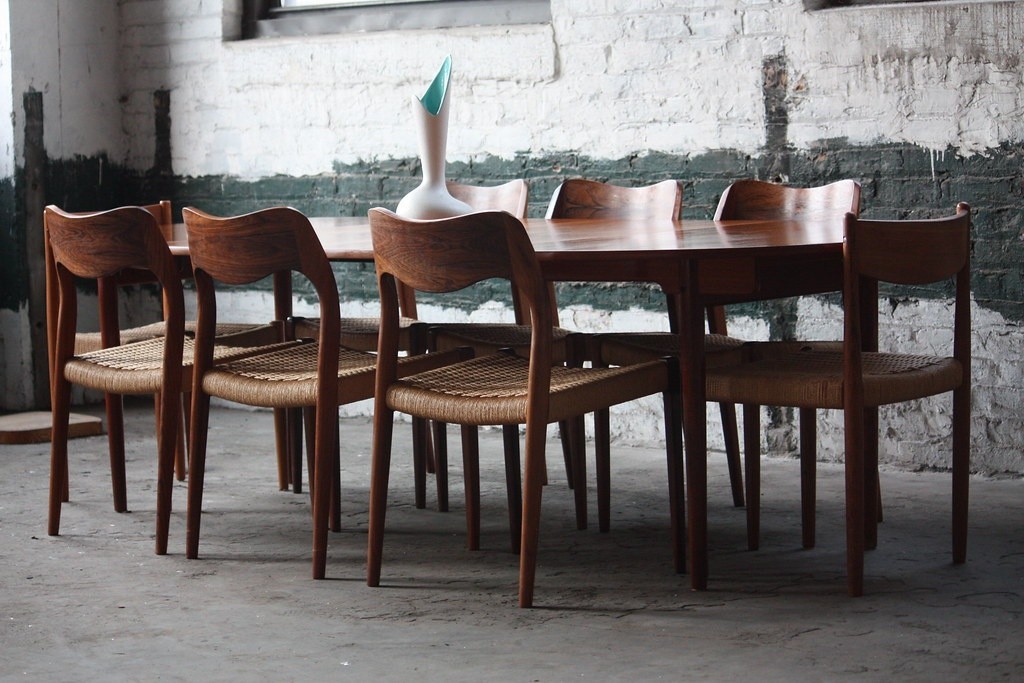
[{"x1": 396, "y1": 53, "x2": 472, "y2": 222}]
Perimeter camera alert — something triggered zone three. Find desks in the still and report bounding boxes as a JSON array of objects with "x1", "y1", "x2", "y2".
[{"x1": 94, "y1": 220, "x2": 879, "y2": 594}]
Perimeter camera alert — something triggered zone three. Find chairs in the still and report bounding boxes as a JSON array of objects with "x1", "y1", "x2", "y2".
[
  {"x1": 594, "y1": 178, "x2": 861, "y2": 549},
  {"x1": 431, "y1": 180, "x2": 682, "y2": 530},
  {"x1": 47, "y1": 206, "x2": 316, "y2": 555},
  {"x1": 182, "y1": 206, "x2": 475, "y2": 580},
  {"x1": 690, "y1": 200, "x2": 973, "y2": 597},
  {"x1": 368, "y1": 206, "x2": 687, "y2": 608},
  {"x1": 288, "y1": 180, "x2": 527, "y2": 510},
  {"x1": 44, "y1": 199, "x2": 285, "y2": 503}
]
